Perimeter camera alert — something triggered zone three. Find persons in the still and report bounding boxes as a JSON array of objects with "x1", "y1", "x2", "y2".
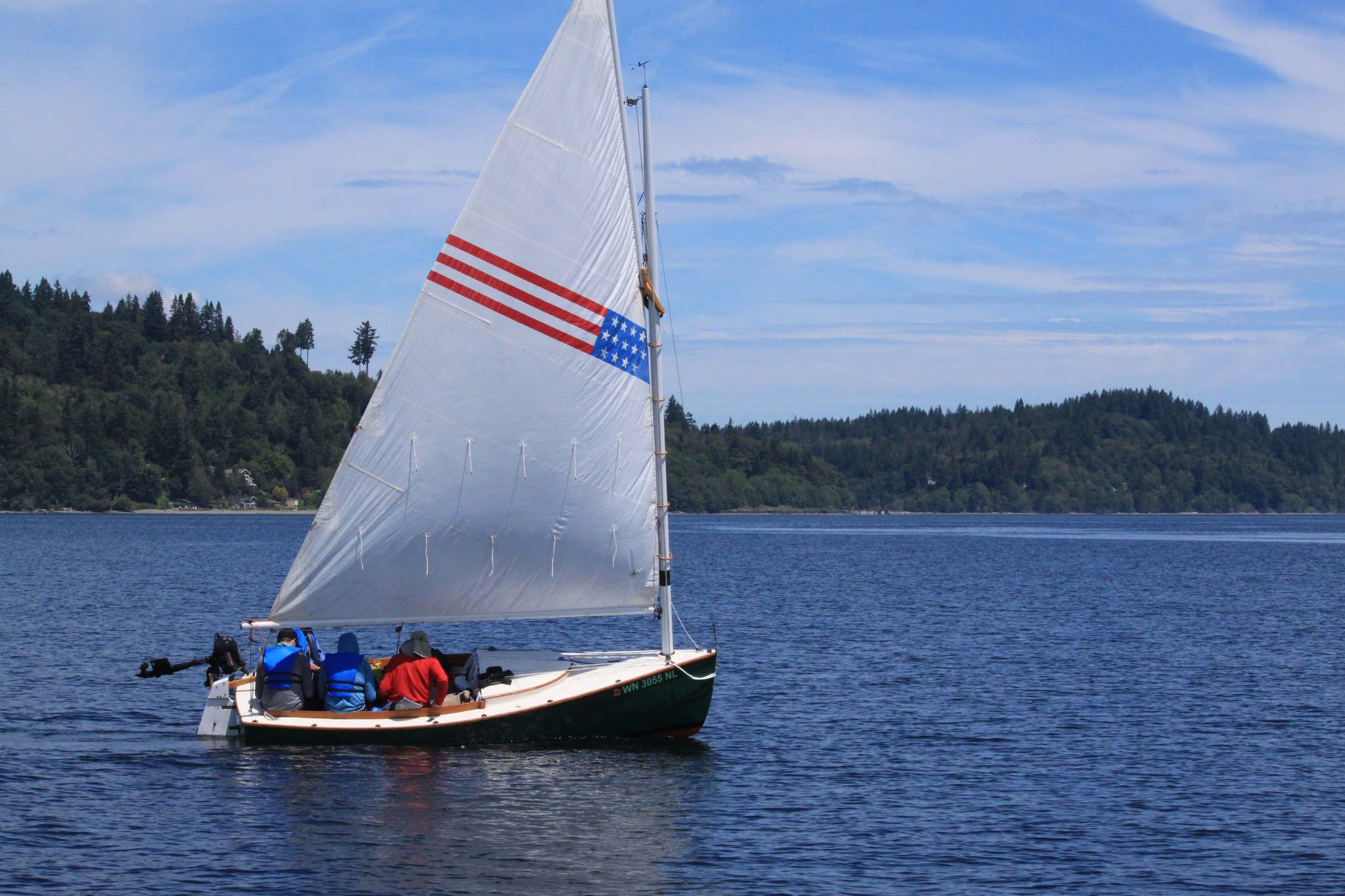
[
  {"x1": 291, "y1": 627, "x2": 325, "y2": 671},
  {"x1": 378, "y1": 631, "x2": 448, "y2": 708},
  {"x1": 318, "y1": 633, "x2": 376, "y2": 713},
  {"x1": 255, "y1": 628, "x2": 314, "y2": 712}
]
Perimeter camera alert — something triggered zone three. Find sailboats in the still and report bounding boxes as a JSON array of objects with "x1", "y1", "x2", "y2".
[{"x1": 128, "y1": 0, "x2": 721, "y2": 752}]
